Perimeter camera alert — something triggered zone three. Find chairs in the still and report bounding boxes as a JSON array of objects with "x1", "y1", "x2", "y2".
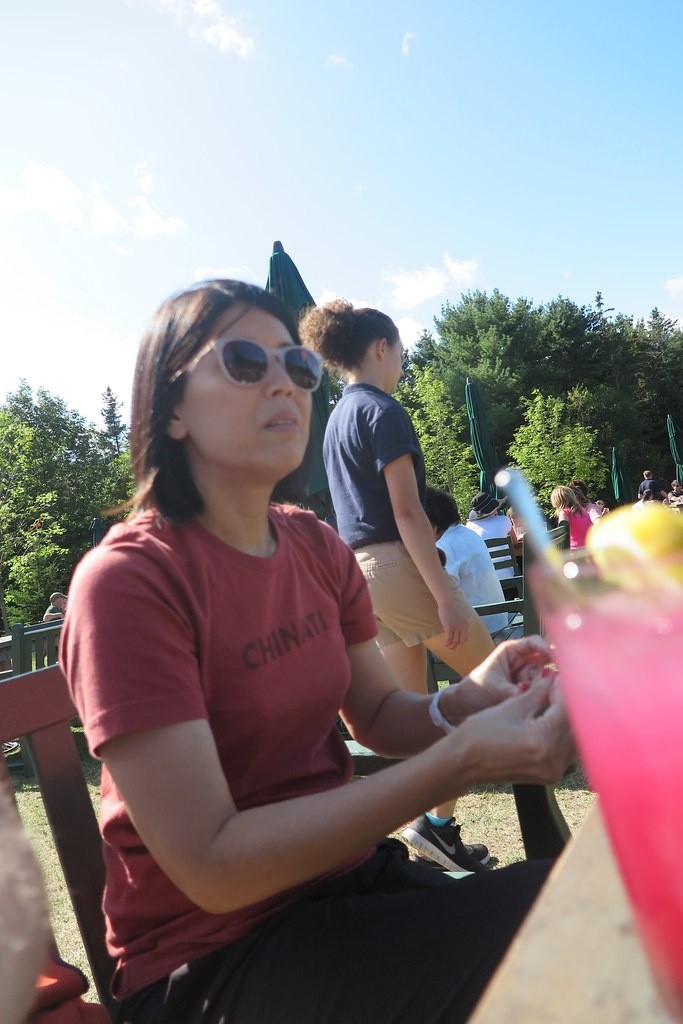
[
  {"x1": 0, "y1": 617, "x2": 576, "y2": 1018},
  {"x1": 427, "y1": 521, "x2": 569, "y2": 695}
]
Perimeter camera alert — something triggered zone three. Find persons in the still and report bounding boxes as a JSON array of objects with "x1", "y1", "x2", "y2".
[
  {"x1": 633, "y1": 470, "x2": 683, "y2": 509},
  {"x1": 59, "y1": 279, "x2": 576, "y2": 1024},
  {"x1": 417, "y1": 481, "x2": 610, "y2": 645},
  {"x1": 42, "y1": 593, "x2": 68, "y2": 666},
  {"x1": 297, "y1": 297, "x2": 497, "y2": 874}
]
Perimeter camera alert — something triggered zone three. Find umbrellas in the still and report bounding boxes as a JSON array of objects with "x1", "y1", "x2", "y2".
[
  {"x1": 611, "y1": 447, "x2": 636, "y2": 504},
  {"x1": 465, "y1": 377, "x2": 509, "y2": 516},
  {"x1": 262, "y1": 241, "x2": 344, "y2": 520},
  {"x1": 667, "y1": 414, "x2": 683, "y2": 485}
]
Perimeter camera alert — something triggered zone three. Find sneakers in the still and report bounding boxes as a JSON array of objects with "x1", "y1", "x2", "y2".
[
  {"x1": 412, "y1": 843, "x2": 490, "y2": 872},
  {"x1": 402, "y1": 812, "x2": 491, "y2": 872}
]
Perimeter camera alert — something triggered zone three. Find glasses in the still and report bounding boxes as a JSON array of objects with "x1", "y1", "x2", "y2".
[
  {"x1": 172, "y1": 338, "x2": 324, "y2": 392},
  {"x1": 674, "y1": 485, "x2": 682, "y2": 487}
]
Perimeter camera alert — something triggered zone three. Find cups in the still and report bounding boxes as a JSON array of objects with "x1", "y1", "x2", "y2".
[{"x1": 525, "y1": 546, "x2": 682, "y2": 1024}]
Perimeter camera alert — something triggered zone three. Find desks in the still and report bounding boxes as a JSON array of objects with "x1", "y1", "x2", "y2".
[
  {"x1": 0, "y1": 625, "x2": 61, "y2": 672},
  {"x1": 465, "y1": 795, "x2": 683, "y2": 1024}
]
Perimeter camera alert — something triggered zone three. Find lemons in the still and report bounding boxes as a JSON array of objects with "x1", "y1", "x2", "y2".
[{"x1": 584, "y1": 502, "x2": 683, "y2": 593}]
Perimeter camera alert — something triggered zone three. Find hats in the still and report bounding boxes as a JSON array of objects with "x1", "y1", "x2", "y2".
[
  {"x1": 50, "y1": 592, "x2": 67, "y2": 602},
  {"x1": 469, "y1": 492, "x2": 507, "y2": 520}
]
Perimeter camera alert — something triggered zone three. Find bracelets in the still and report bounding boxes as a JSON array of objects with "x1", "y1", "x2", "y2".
[{"x1": 429, "y1": 685, "x2": 457, "y2": 736}]
